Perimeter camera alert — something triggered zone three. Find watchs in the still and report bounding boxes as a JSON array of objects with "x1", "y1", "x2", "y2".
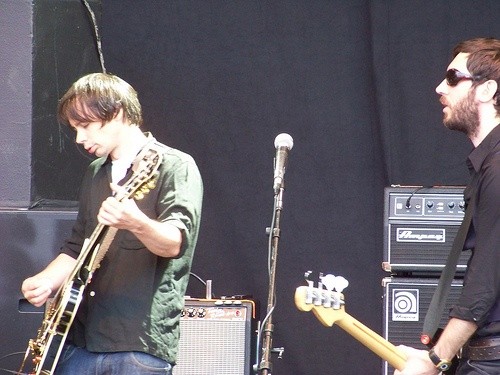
[{"x1": 429, "y1": 350, "x2": 453, "y2": 373}]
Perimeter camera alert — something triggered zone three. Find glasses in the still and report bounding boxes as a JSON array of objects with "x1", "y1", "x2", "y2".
[{"x1": 446, "y1": 69, "x2": 482, "y2": 86}]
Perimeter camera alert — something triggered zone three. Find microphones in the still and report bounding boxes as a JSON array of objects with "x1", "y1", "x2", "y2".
[{"x1": 273, "y1": 132, "x2": 294, "y2": 194}]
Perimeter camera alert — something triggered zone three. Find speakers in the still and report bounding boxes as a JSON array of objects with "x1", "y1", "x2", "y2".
[
  {"x1": 0, "y1": 204, "x2": 80, "y2": 374},
  {"x1": 168, "y1": 300, "x2": 253, "y2": 375},
  {"x1": 382, "y1": 277, "x2": 464, "y2": 375}
]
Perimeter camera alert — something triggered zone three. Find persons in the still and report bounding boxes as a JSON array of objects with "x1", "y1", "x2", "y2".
[
  {"x1": 390, "y1": 38, "x2": 500, "y2": 375},
  {"x1": 21, "y1": 72, "x2": 205, "y2": 375}
]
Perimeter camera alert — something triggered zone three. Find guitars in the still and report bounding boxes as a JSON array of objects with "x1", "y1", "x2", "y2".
[
  {"x1": 295, "y1": 270, "x2": 408, "y2": 371},
  {"x1": 31, "y1": 153, "x2": 163, "y2": 375}
]
